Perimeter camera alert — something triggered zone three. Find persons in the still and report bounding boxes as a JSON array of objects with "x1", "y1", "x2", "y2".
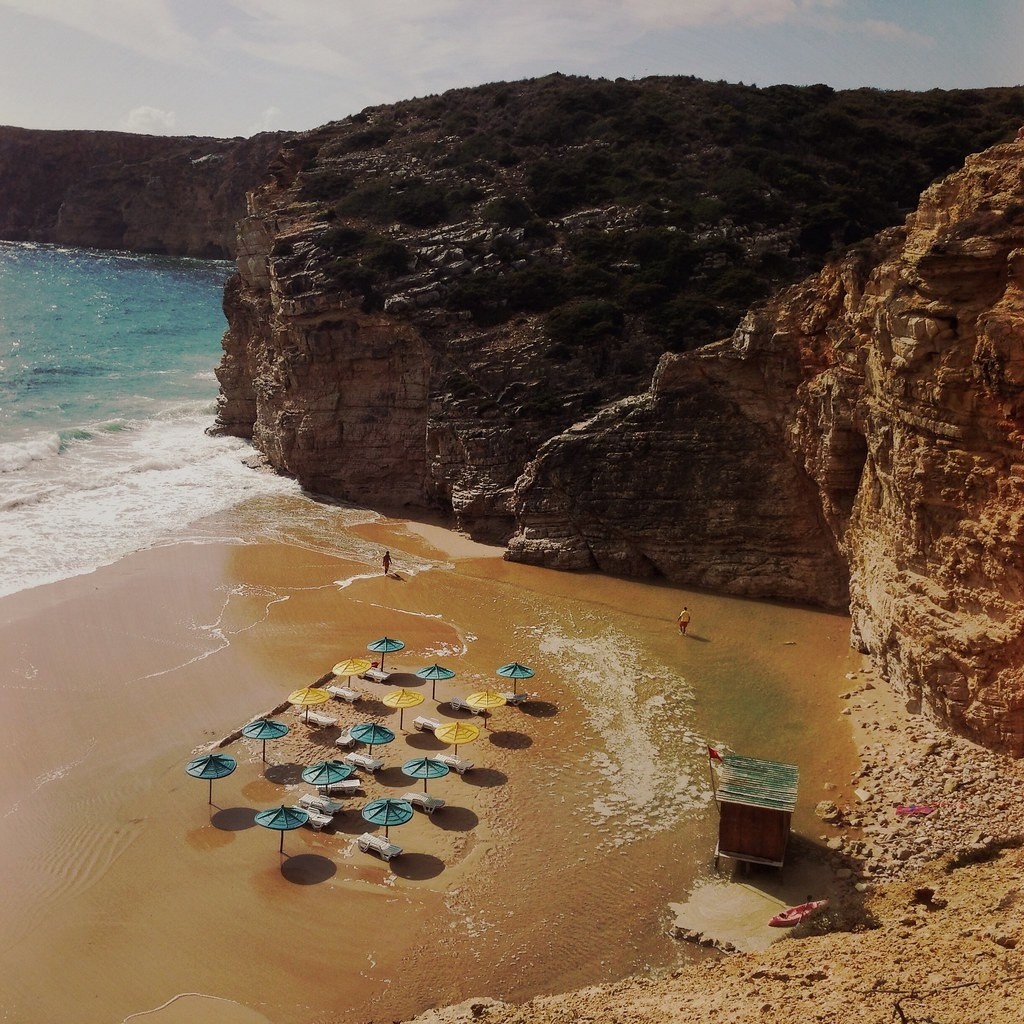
[
  {"x1": 676, "y1": 607, "x2": 690, "y2": 634},
  {"x1": 383, "y1": 551, "x2": 392, "y2": 574}
]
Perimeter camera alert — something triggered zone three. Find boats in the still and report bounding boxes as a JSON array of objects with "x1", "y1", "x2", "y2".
[{"x1": 768, "y1": 899, "x2": 829, "y2": 926}]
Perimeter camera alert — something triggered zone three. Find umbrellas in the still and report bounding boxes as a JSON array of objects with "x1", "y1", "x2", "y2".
[
  {"x1": 496, "y1": 661, "x2": 535, "y2": 694},
  {"x1": 382, "y1": 689, "x2": 426, "y2": 730},
  {"x1": 289, "y1": 687, "x2": 331, "y2": 726},
  {"x1": 242, "y1": 718, "x2": 289, "y2": 762},
  {"x1": 332, "y1": 657, "x2": 372, "y2": 687},
  {"x1": 254, "y1": 804, "x2": 309, "y2": 853},
  {"x1": 433, "y1": 721, "x2": 480, "y2": 755},
  {"x1": 367, "y1": 637, "x2": 405, "y2": 673},
  {"x1": 416, "y1": 664, "x2": 456, "y2": 699},
  {"x1": 362, "y1": 798, "x2": 413, "y2": 838},
  {"x1": 185, "y1": 753, "x2": 237, "y2": 805},
  {"x1": 466, "y1": 689, "x2": 507, "y2": 728},
  {"x1": 301, "y1": 761, "x2": 352, "y2": 796},
  {"x1": 350, "y1": 723, "x2": 395, "y2": 754},
  {"x1": 401, "y1": 756, "x2": 450, "y2": 793}
]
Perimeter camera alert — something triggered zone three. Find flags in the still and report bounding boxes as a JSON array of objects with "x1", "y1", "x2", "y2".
[{"x1": 707, "y1": 744, "x2": 724, "y2": 763}]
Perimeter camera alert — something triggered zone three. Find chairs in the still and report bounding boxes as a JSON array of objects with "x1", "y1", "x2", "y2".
[
  {"x1": 291, "y1": 805, "x2": 333, "y2": 830},
  {"x1": 327, "y1": 686, "x2": 364, "y2": 704},
  {"x1": 297, "y1": 794, "x2": 344, "y2": 815},
  {"x1": 345, "y1": 752, "x2": 385, "y2": 774},
  {"x1": 433, "y1": 754, "x2": 474, "y2": 774},
  {"x1": 499, "y1": 692, "x2": 528, "y2": 705},
  {"x1": 413, "y1": 716, "x2": 449, "y2": 730},
  {"x1": 357, "y1": 832, "x2": 403, "y2": 861},
  {"x1": 300, "y1": 711, "x2": 337, "y2": 727},
  {"x1": 315, "y1": 779, "x2": 360, "y2": 797},
  {"x1": 450, "y1": 696, "x2": 488, "y2": 714},
  {"x1": 335, "y1": 727, "x2": 358, "y2": 747},
  {"x1": 401, "y1": 792, "x2": 446, "y2": 813},
  {"x1": 362, "y1": 666, "x2": 391, "y2": 682}
]
